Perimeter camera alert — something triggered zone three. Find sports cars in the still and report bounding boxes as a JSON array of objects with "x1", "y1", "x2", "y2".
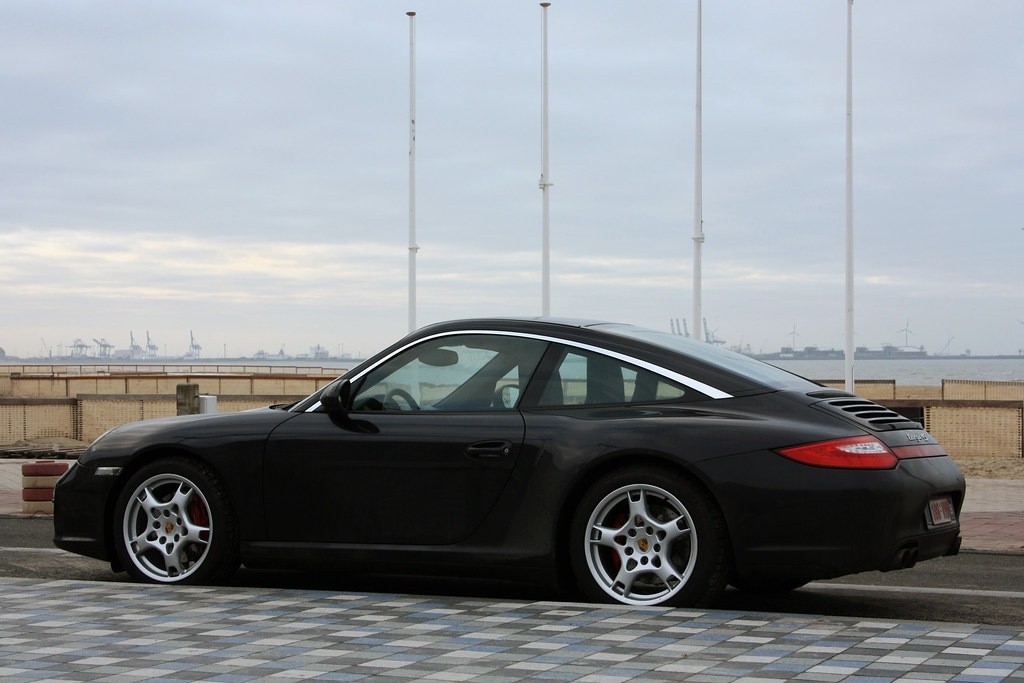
[{"x1": 50, "y1": 314, "x2": 968, "y2": 617}]
